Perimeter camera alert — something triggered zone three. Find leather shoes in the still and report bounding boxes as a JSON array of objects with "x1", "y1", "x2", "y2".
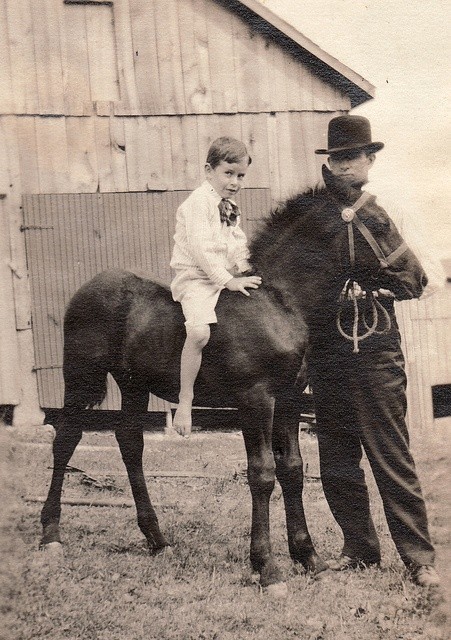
[
  {"x1": 325, "y1": 553, "x2": 380, "y2": 570},
  {"x1": 416, "y1": 566, "x2": 438, "y2": 586}
]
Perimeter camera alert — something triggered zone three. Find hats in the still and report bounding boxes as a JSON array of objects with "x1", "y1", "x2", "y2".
[{"x1": 315, "y1": 115, "x2": 383, "y2": 154}]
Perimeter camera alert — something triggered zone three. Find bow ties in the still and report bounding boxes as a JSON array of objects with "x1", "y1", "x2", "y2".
[{"x1": 219, "y1": 199, "x2": 239, "y2": 226}]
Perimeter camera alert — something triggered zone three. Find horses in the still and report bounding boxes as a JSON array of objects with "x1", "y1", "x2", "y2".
[{"x1": 40, "y1": 164, "x2": 428, "y2": 596}]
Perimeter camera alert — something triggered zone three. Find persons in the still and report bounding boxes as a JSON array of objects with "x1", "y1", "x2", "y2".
[
  {"x1": 171, "y1": 138, "x2": 260, "y2": 439},
  {"x1": 315, "y1": 115, "x2": 436, "y2": 584}
]
